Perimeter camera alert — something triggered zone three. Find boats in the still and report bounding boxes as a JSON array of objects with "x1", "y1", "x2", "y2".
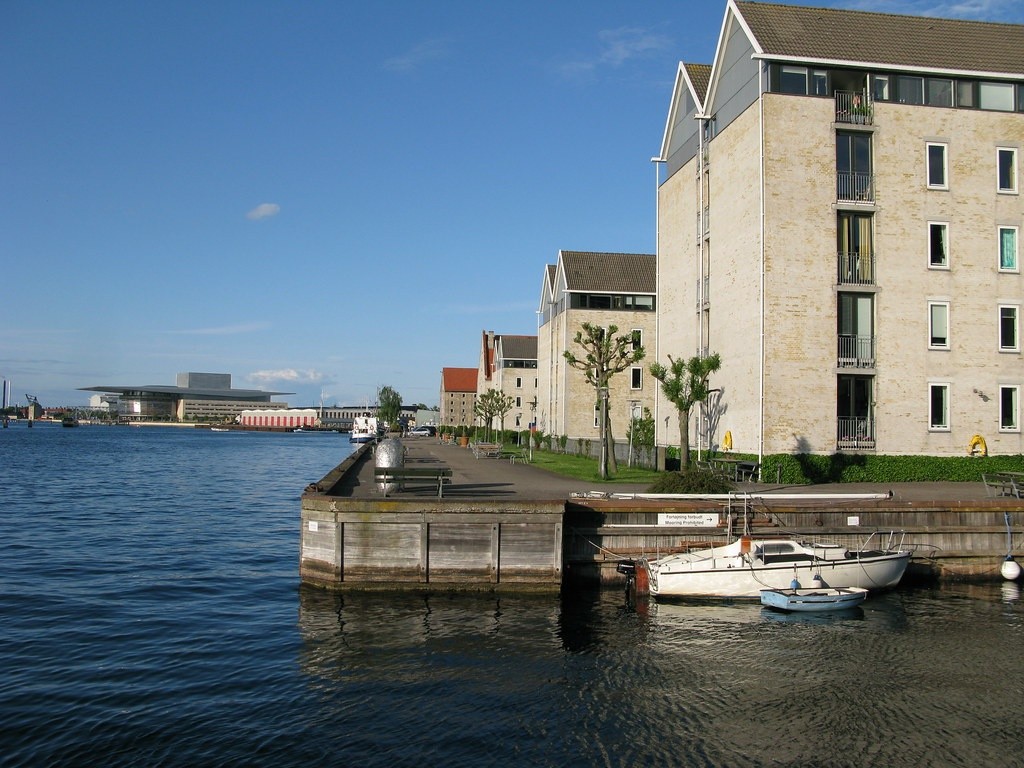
[
  {"x1": 210, "y1": 428, "x2": 230, "y2": 432},
  {"x1": 758, "y1": 585, "x2": 870, "y2": 613},
  {"x1": 349, "y1": 411, "x2": 379, "y2": 443},
  {"x1": 615, "y1": 529, "x2": 914, "y2": 603},
  {"x1": 62, "y1": 417, "x2": 80, "y2": 428},
  {"x1": 295, "y1": 429, "x2": 340, "y2": 434}
]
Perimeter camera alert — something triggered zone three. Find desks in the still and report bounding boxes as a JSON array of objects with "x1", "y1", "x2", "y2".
[
  {"x1": 403, "y1": 454, "x2": 440, "y2": 463},
  {"x1": 712, "y1": 459, "x2": 742, "y2": 482},
  {"x1": 404, "y1": 464, "x2": 450, "y2": 495},
  {"x1": 997, "y1": 471, "x2": 1024, "y2": 498}
]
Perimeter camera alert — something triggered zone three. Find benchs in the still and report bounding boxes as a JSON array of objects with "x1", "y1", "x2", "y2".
[
  {"x1": 695, "y1": 461, "x2": 723, "y2": 480},
  {"x1": 731, "y1": 461, "x2": 759, "y2": 482},
  {"x1": 374, "y1": 466, "x2": 453, "y2": 498},
  {"x1": 982, "y1": 474, "x2": 1024, "y2": 499}
]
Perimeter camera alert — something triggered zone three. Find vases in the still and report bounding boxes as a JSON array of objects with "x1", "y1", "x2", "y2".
[{"x1": 836, "y1": 115, "x2": 851, "y2": 122}]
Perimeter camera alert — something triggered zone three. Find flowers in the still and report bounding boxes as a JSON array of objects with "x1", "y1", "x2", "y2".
[{"x1": 837, "y1": 109, "x2": 850, "y2": 116}]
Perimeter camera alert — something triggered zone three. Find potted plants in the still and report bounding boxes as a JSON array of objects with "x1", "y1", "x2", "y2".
[
  {"x1": 856, "y1": 106, "x2": 865, "y2": 125},
  {"x1": 865, "y1": 105, "x2": 873, "y2": 125},
  {"x1": 849, "y1": 107, "x2": 857, "y2": 124}
]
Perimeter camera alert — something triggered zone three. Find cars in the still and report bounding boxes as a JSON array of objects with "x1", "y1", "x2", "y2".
[{"x1": 407, "y1": 426, "x2": 435, "y2": 437}]
